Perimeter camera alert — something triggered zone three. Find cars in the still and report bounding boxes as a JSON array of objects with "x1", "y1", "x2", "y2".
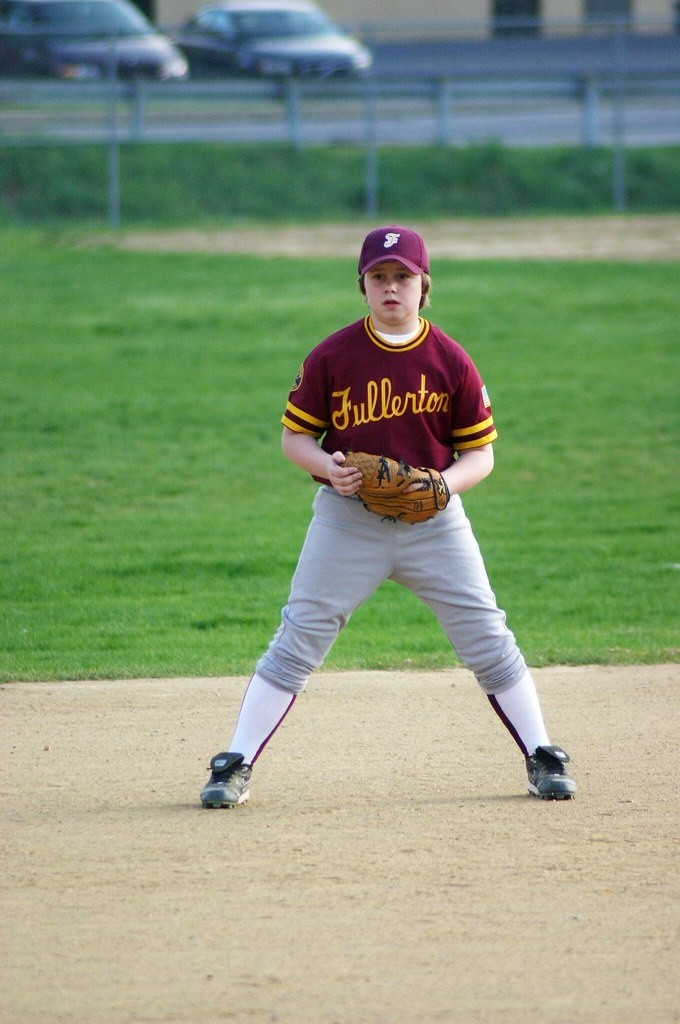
[
  {"x1": 0, "y1": 0, "x2": 194, "y2": 90},
  {"x1": 165, "y1": 0, "x2": 373, "y2": 97}
]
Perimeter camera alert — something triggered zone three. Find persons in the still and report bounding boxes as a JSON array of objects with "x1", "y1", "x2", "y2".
[{"x1": 198, "y1": 228, "x2": 578, "y2": 812}]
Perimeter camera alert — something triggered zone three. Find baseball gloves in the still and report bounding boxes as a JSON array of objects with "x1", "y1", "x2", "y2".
[{"x1": 340, "y1": 449, "x2": 451, "y2": 527}]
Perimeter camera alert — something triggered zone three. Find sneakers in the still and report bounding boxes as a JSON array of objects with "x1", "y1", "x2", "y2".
[
  {"x1": 526, "y1": 745, "x2": 576, "y2": 800},
  {"x1": 200, "y1": 752, "x2": 253, "y2": 808}
]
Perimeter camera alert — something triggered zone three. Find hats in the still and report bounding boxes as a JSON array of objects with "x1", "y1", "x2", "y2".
[{"x1": 358, "y1": 224, "x2": 429, "y2": 275}]
